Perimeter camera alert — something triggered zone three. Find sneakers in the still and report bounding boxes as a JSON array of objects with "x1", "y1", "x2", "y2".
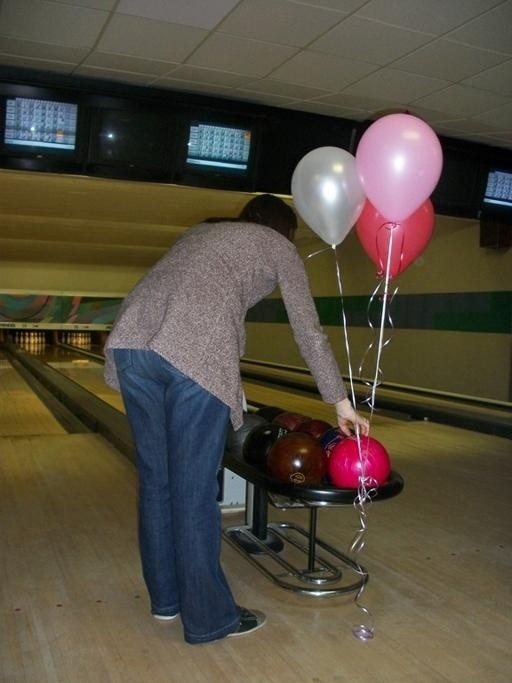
[{"x1": 223, "y1": 609, "x2": 267, "y2": 637}]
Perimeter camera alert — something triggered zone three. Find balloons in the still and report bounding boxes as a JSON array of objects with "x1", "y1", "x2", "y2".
[
  {"x1": 356, "y1": 113, "x2": 444, "y2": 230},
  {"x1": 356, "y1": 196, "x2": 435, "y2": 277},
  {"x1": 291, "y1": 146, "x2": 365, "y2": 249}
]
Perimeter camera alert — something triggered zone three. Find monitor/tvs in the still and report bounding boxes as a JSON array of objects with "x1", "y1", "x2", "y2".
[
  {"x1": 184, "y1": 119, "x2": 255, "y2": 176},
  {"x1": 0, "y1": 83, "x2": 90, "y2": 157},
  {"x1": 481, "y1": 168, "x2": 512, "y2": 209}
]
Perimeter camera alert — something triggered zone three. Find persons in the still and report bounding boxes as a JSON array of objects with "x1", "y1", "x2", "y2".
[{"x1": 102, "y1": 194, "x2": 370, "y2": 644}]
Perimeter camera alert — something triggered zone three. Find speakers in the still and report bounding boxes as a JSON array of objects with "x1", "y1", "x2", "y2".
[{"x1": 479, "y1": 213, "x2": 512, "y2": 250}]
